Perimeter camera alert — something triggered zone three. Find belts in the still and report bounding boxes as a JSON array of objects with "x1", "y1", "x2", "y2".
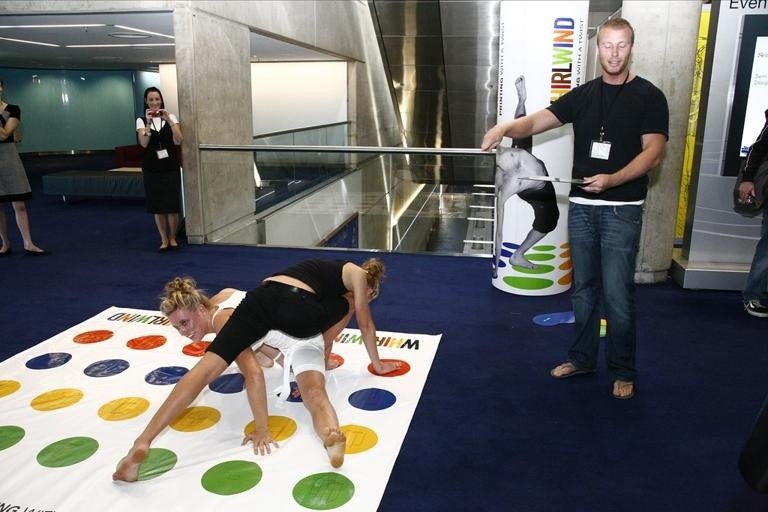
[{"x1": 262, "y1": 280, "x2": 318, "y2": 300}]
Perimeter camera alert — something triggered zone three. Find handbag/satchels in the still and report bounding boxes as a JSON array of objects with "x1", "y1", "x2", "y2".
[{"x1": 733, "y1": 155, "x2": 768, "y2": 217}]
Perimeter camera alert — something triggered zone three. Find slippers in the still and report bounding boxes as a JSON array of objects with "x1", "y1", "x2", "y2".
[
  {"x1": 550, "y1": 361, "x2": 597, "y2": 379},
  {"x1": 612, "y1": 379, "x2": 635, "y2": 399}
]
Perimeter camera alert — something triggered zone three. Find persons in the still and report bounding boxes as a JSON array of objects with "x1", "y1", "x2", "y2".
[
  {"x1": 160, "y1": 279, "x2": 346, "y2": 469},
  {"x1": 0, "y1": 80, "x2": 46, "y2": 256},
  {"x1": 495, "y1": 74, "x2": 560, "y2": 278},
  {"x1": 135, "y1": 87, "x2": 183, "y2": 253},
  {"x1": 482, "y1": 19, "x2": 669, "y2": 400},
  {"x1": 113, "y1": 257, "x2": 400, "y2": 484},
  {"x1": 738, "y1": 109, "x2": 768, "y2": 317}
]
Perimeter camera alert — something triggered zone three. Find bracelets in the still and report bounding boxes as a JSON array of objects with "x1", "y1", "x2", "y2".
[{"x1": 142, "y1": 133, "x2": 152, "y2": 137}]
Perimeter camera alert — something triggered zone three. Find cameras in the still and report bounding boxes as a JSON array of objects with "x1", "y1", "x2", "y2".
[{"x1": 149, "y1": 110, "x2": 160, "y2": 117}]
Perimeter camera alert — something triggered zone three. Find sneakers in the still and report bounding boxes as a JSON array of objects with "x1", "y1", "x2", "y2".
[
  {"x1": 24, "y1": 246, "x2": 48, "y2": 256},
  {"x1": 0, "y1": 245, "x2": 11, "y2": 258},
  {"x1": 744, "y1": 298, "x2": 767, "y2": 319},
  {"x1": 158, "y1": 241, "x2": 178, "y2": 252}
]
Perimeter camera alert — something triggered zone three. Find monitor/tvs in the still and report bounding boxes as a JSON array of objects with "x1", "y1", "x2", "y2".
[{"x1": 735, "y1": 32, "x2": 768, "y2": 161}]
